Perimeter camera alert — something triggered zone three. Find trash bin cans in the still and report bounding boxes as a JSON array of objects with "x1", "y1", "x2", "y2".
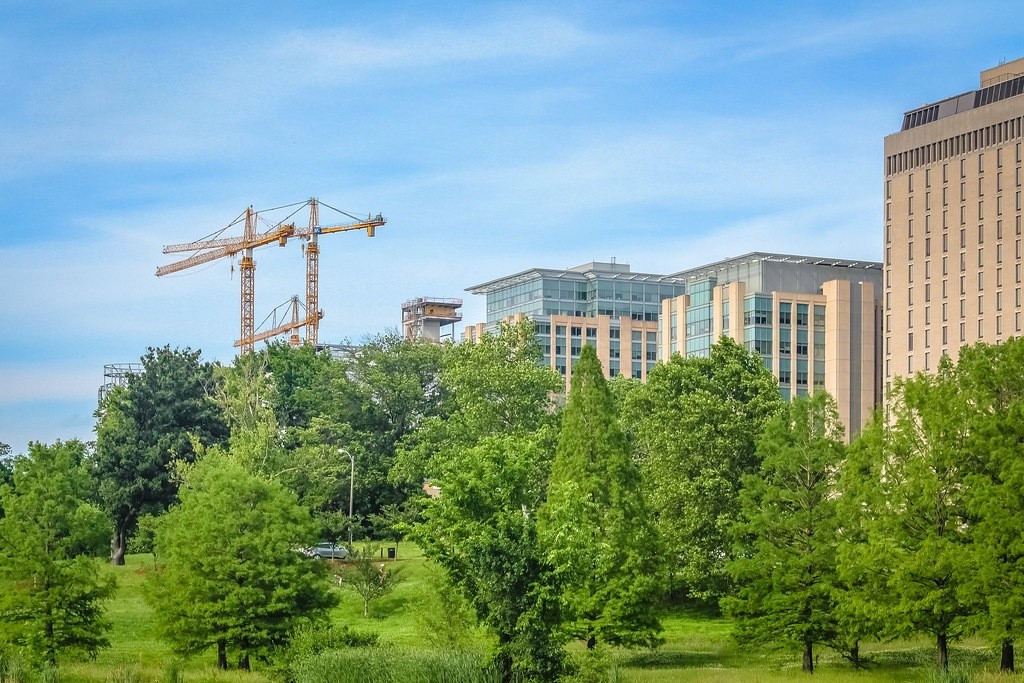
[{"x1": 388, "y1": 548, "x2": 395, "y2": 558}]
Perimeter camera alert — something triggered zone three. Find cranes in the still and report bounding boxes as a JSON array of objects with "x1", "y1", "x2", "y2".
[
  {"x1": 161, "y1": 198, "x2": 388, "y2": 346},
  {"x1": 157, "y1": 206, "x2": 296, "y2": 358},
  {"x1": 233, "y1": 297, "x2": 324, "y2": 350}
]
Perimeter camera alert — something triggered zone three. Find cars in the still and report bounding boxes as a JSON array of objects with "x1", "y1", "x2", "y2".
[{"x1": 308, "y1": 542, "x2": 353, "y2": 561}]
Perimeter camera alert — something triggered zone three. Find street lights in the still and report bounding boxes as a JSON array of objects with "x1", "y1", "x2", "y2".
[{"x1": 338, "y1": 449, "x2": 355, "y2": 549}]
